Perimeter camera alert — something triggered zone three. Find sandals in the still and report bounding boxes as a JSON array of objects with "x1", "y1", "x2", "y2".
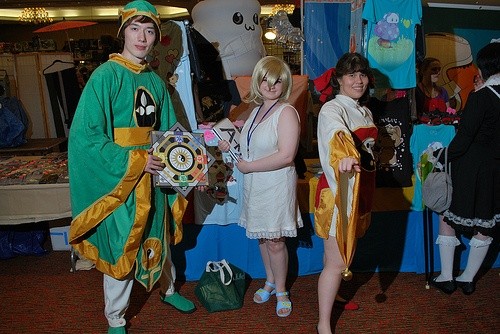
[
  {"x1": 275, "y1": 290, "x2": 292, "y2": 317},
  {"x1": 253, "y1": 281, "x2": 276, "y2": 303}
]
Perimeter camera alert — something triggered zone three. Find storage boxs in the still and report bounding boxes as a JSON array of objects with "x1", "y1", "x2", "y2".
[{"x1": 49, "y1": 217, "x2": 72, "y2": 251}]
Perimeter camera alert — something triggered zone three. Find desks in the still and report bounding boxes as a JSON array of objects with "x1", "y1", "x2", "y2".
[
  {"x1": 167, "y1": 160, "x2": 500, "y2": 282},
  {"x1": 0, "y1": 138, "x2": 67, "y2": 156}
]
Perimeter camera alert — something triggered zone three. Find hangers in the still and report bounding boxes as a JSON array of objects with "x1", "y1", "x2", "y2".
[{"x1": 42, "y1": 59, "x2": 76, "y2": 75}]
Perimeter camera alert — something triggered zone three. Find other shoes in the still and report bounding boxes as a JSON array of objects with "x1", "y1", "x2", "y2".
[
  {"x1": 108, "y1": 327, "x2": 126, "y2": 334},
  {"x1": 159, "y1": 290, "x2": 195, "y2": 314},
  {"x1": 431, "y1": 275, "x2": 456, "y2": 296},
  {"x1": 454, "y1": 276, "x2": 475, "y2": 294},
  {"x1": 333, "y1": 299, "x2": 359, "y2": 310}
]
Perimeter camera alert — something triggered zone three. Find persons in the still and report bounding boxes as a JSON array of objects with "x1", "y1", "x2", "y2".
[
  {"x1": 68, "y1": 0, "x2": 210, "y2": 334},
  {"x1": 218, "y1": 57, "x2": 304, "y2": 318},
  {"x1": 428, "y1": 42, "x2": 500, "y2": 295},
  {"x1": 416, "y1": 56, "x2": 450, "y2": 116},
  {"x1": 314, "y1": 53, "x2": 378, "y2": 334}
]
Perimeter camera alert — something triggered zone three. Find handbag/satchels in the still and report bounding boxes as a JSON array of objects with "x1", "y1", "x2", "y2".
[
  {"x1": 194, "y1": 259, "x2": 252, "y2": 313},
  {"x1": 422, "y1": 147, "x2": 453, "y2": 214}
]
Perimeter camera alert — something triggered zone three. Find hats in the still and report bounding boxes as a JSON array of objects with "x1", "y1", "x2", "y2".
[{"x1": 117, "y1": 0, "x2": 162, "y2": 42}]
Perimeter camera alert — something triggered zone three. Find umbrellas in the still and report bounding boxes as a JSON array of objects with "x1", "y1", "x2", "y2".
[{"x1": 33, "y1": 17, "x2": 98, "y2": 51}]
